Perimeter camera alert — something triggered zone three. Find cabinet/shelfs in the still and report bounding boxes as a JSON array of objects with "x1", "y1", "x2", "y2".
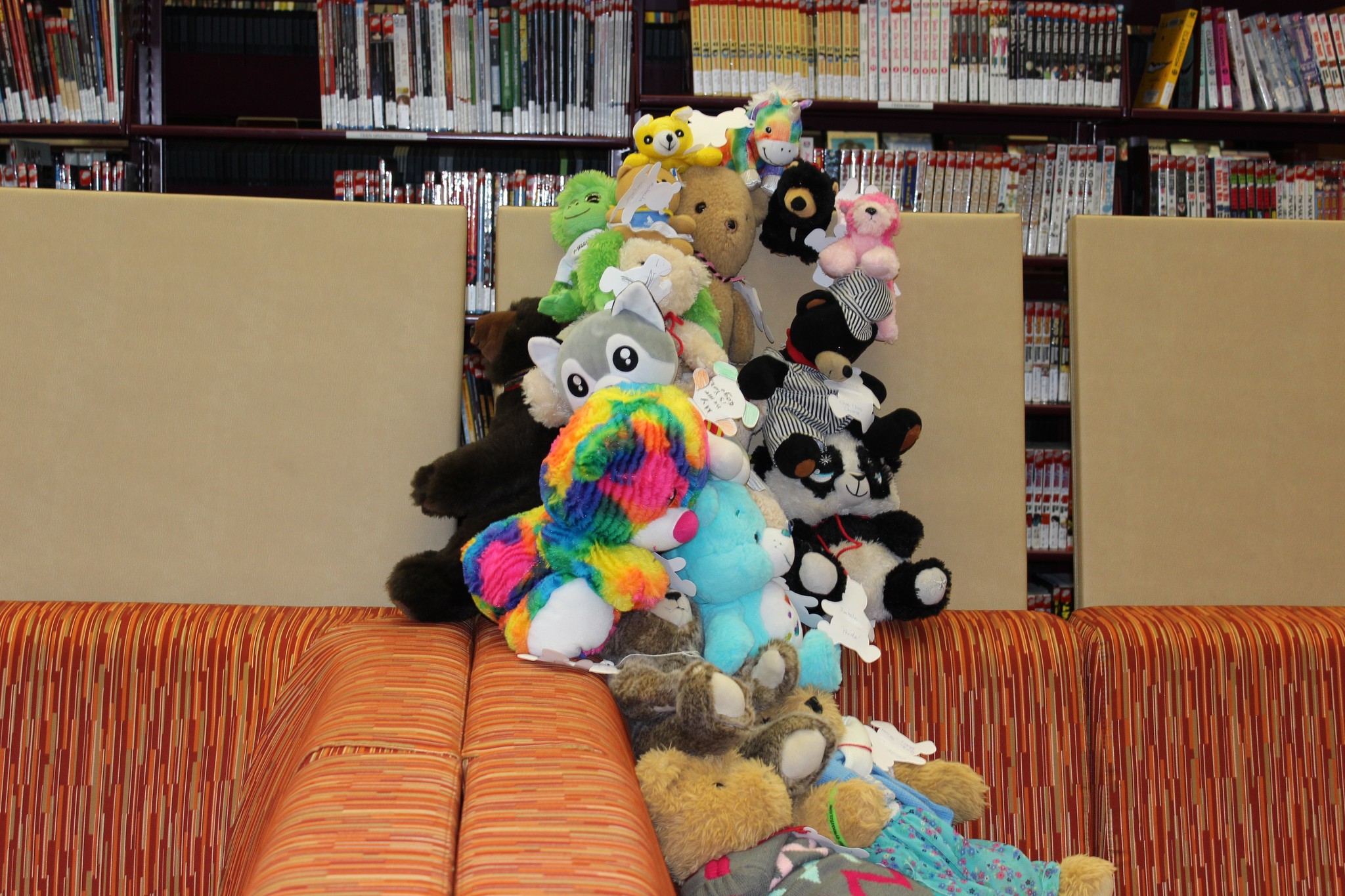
[{"x1": 0, "y1": 0, "x2": 1345, "y2": 556}]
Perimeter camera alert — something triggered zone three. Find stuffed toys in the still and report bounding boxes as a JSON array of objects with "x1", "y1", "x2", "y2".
[{"x1": 382, "y1": 90, "x2": 1118, "y2": 896}]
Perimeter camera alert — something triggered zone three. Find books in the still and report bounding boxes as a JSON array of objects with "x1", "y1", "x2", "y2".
[
  {"x1": 317, "y1": 1, "x2": 634, "y2": 316},
  {"x1": 637, "y1": 2, "x2": 1345, "y2": 616},
  {"x1": 0, "y1": 0, "x2": 125, "y2": 191}
]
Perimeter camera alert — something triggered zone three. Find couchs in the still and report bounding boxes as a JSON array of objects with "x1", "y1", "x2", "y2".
[
  {"x1": 466, "y1": 606, "x2": 1345, "y2": 896},
  {"x1": 0, "y1": 598, "x2": 472, "y2": 896}
]
